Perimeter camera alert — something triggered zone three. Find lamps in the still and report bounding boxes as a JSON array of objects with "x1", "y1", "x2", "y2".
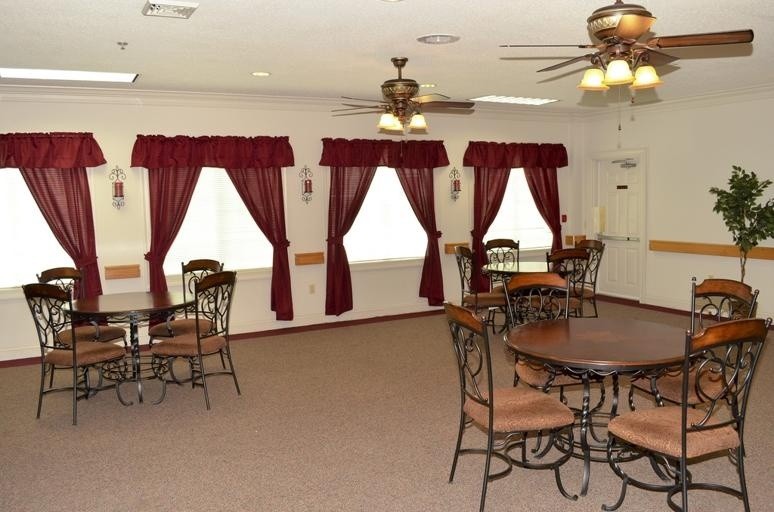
[
  {"x1": 105, "y1": 162, "x2": 129, "y2": 212},
  {"x1": 448, "y1": 167, "x2": 463, "y2": 203},
  {"x1": 296, "y1": 163, "x2": 314, "y2": 206},
  {"x1": 575, "y1": 51, "x2": 662, "y2": 95},
  {"x1": 378, "y1": 100, "x2": 431, "y2": 133}
]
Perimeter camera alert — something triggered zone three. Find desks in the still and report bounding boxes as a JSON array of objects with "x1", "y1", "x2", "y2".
[{"x1": 58, "y1": 286, "x2": 204, "y2": 404}]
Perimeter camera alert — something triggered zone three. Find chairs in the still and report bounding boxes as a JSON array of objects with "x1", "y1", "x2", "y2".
[
  {"x1": 20, "y1": 280, "x2": 137, "y2": 425},
  {"x1": 151, "y1": 258, "x2": 227, "y2": 375},
  {"x1": 38, "y1": 267, "x2": 131, "y2": 395},
  {"x1": 144, "y1": 270, "x2": 239, "y2": 410}
]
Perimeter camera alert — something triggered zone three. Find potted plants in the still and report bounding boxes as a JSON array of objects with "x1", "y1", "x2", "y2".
[{"x1": 707, "y1": 162, "x2": 772, "y2": 322}]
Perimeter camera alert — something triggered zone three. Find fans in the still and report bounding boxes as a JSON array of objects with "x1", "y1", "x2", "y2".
[
  {"x1": 328, "y1": 56, "x2": 481, "y2": 117},
  {"x1": 493, "y1": 3, "x2": 758, "y2": 74}
]
